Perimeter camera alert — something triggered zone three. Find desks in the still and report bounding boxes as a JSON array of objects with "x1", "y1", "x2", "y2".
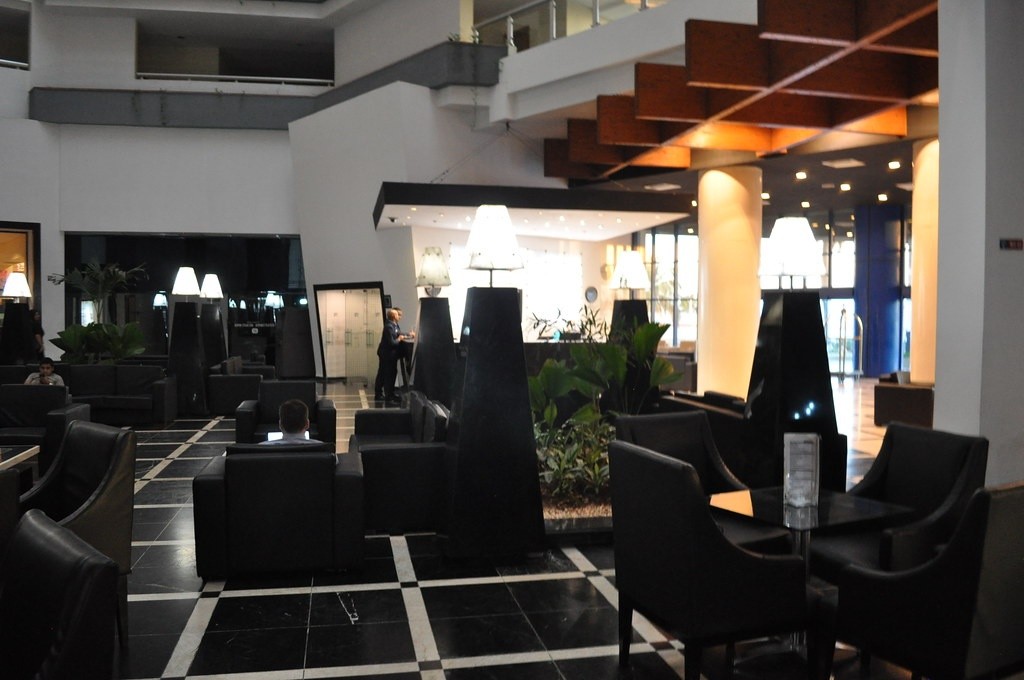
[
  {"x1": 0, "y1": 444, "x2": 41, "y2": 472},
  {"x1": 709, "y1": 486, "x2": 916, "y2": 679}
]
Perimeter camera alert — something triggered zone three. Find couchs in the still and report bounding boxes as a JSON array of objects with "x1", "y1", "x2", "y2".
[
  {"x1": 193, "y1": 442, "x2": 365, "y2": 581},
  {"x1": 662, "y1": 385, "x2": 850, "y2": 489},
  {"x1": 208, "y1": 353, "x2": 275, "y2": 410},
  {"x1": 0, "y1": 382, "x2": 89, "y2": 458},
  {"x1": 0, "y1": 365, "x2": 180, "y2": 426},
  {"x1": 351, "y1": 401, "x2": 452, "y2": 534},
  {"x1": 234, "y1": 380, "x2": 338, "y2": 444}
]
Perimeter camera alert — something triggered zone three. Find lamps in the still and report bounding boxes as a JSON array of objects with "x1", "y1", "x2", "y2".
[
  {"x1": 416, "y1": 246, "x2": 452, "y2": 298},
  {"x1": 467, "y1": 204, "x2": 522, "y2": 288},
  {"x1": 199, "y1": 273, "x2": 222, "y2": 299},
  {"x1": 171, "y1": 267, "x2": 198, "y2": 295},
  {"x1": 606, "y1": 250, "x2": 650, "y2": 299},
  {"x1": 1, "y1": 271, "x2": 33, "y2": 303},
  {"x1": 757, "y1": 213, "x2": 829, "y2": 288}
]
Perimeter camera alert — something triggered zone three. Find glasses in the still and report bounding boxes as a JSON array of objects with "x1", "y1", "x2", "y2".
[{"x1": 40, "y1": 366, "x2": 52, "y2": 370}]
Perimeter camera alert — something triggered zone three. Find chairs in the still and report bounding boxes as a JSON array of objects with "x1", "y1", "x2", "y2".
[
  {"x1": 606, "y1": 411, "x2": 1024, "y2": 680},
  {"x1": 0, "y1": 421, "x2": 135, "y2": 679}
]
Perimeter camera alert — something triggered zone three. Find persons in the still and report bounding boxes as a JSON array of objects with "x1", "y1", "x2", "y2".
[
  {"x1": 259, "y1": 399, "x2": 339, "y2": 465},
  {"x1": 375, "y1": 308, "x2": 414, "y2": 405},
  {"x1": 24, "y1": 357, "x2": 64, "y2": 386},
  {"x1": 23, "y1": 309, "x2": 44, "y2": 363}
]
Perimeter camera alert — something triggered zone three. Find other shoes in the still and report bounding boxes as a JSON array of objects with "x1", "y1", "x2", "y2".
[
  {"x1": 384, "y1": 396, "x2": 401, "y2": 403},
  {"x1": 374, "y1": 394, "x2": 385, "y2": 401}
]
